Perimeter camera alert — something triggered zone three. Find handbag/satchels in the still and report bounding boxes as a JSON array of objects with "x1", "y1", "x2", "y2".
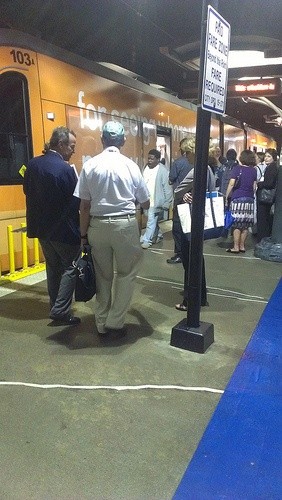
[
  {"x1": 177, "y1": 170, "x2": 225, "y2": 234},
  {"x1": 72, "y1": 247, "x2": 95, "y2": 302},
  {"x1": 224, "y1": 209, "x2": 235, "y2": 230},
  {"x1": 258, "y1": 187, "x2": 276, "y2": 204},
  {"x1": 233, "y1": 166, "x2": 242, "y2": 188}
]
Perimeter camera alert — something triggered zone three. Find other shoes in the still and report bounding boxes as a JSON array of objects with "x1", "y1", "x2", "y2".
[
  {"x1": 141, "y1": 242, "x2": 150, "y2": 248},
  {"x1": 47, "y1": 315, "x2": 81, "y2": 325},
  {"x1": 104, "y1": 326, "x2": 125, "y2": 336},
  {"x1": 167, "y1": 255, "x2": 181, "y2": 263},
  {"x1": 156, "y1": 235, "x2": 163, "y2": 243}
]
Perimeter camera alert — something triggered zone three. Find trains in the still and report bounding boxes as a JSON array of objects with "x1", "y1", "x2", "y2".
[{"x1": 0, "y1": 28, "x2": 278, "y2": 274}]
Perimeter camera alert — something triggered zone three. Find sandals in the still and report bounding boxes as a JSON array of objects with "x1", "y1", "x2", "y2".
[{"x1": 175, "y1": 303, "x2": 187, "y2": 311}]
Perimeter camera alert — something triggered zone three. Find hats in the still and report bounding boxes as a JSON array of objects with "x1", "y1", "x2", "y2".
[{"x1": 102, "y1": 121, "x2": 124, "y2": 142}]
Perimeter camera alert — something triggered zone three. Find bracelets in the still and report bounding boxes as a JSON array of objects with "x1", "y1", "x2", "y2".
[{"x1": 81, "y1": 235, "x2": 87, "y2": 239}]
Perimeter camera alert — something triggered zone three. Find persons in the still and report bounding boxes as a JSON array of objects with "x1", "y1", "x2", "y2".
[
  {"x1": 226, "y1": 149, "x2": 257, "y2": 253},
  {"x1": 74, "y1": 121, "x2": 151, "y2": 343},
  {"x1": 168, "y1": 138, "x2": 217, "y2": 311},
  {"x1": 213, "y1": 148, "x2": 277, "y2": 241},
  {"x1": 25, "y1": 125, "x2": 81, "y2": 324},
  {"x1": 143, "y1": 149, "x2": 172, "y2": 249}
]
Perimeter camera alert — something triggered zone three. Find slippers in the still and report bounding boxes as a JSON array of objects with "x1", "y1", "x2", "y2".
[
  {"x1": 226, "y1": 248, "x2": 239, "y2": 253},
  {"x1": 239, "y1": 250, "x2": 245, "y2": 252}
]
